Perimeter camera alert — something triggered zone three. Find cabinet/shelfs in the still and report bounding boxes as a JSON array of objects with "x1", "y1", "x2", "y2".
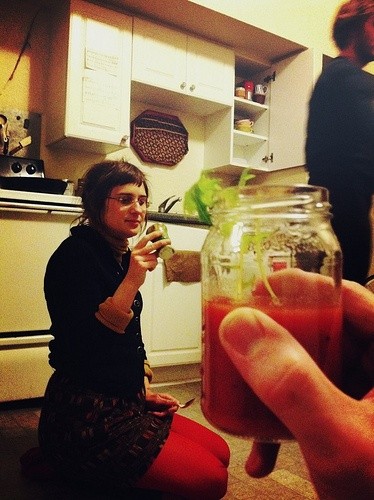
[{"x1": 45, "y1": 1, "x2": 313, "y2": 173}]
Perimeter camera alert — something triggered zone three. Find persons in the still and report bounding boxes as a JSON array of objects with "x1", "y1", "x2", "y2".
[
  {"x1": 305, "y1": 1, "x2": 374, "y2": 288},
  {"x1": 21, "y1": 157, "x2": 232, "y2": 500},
  {"x1": 218, "y1": 267, "x2": 374, "y2": 500}
]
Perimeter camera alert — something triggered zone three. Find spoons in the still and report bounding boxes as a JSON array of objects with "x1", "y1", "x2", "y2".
[{"x1": 177, "y1": 398, "x2": 195, "y2": 409}]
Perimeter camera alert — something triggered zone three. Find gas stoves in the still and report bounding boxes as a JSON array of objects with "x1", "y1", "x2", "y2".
[{"x1": 0, "y1": 154, "x2": 81, "y2": 205}]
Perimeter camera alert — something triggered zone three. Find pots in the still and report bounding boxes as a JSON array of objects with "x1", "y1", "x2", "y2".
[{"x1": 1, "y1": 176, "x2": 68, "y2": 194}]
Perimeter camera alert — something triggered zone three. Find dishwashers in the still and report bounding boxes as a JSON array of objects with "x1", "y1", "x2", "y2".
[{"x1": 0, "y1": 201, "x2": 87, "y2": 402}]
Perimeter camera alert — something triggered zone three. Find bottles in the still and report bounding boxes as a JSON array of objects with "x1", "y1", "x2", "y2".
[{"x1": 202, "y1": 184, "x2": 342, "y2": 443}]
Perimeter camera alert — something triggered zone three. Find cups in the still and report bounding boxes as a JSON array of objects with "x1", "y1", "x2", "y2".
[
  {"x1": 236, "y1": 119, "x2": 254, "y2": 133},
  {"x1": 237, "y1": 81, "x2": 269, "y2": 104},
  {"x1": 146, "y1": 223, "x2": 175, "y2": 261}
]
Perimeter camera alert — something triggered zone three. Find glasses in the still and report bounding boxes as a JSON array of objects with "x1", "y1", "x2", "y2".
[{"x1": 102, "y1": 195, "x2": 147, "y2": 206}]
